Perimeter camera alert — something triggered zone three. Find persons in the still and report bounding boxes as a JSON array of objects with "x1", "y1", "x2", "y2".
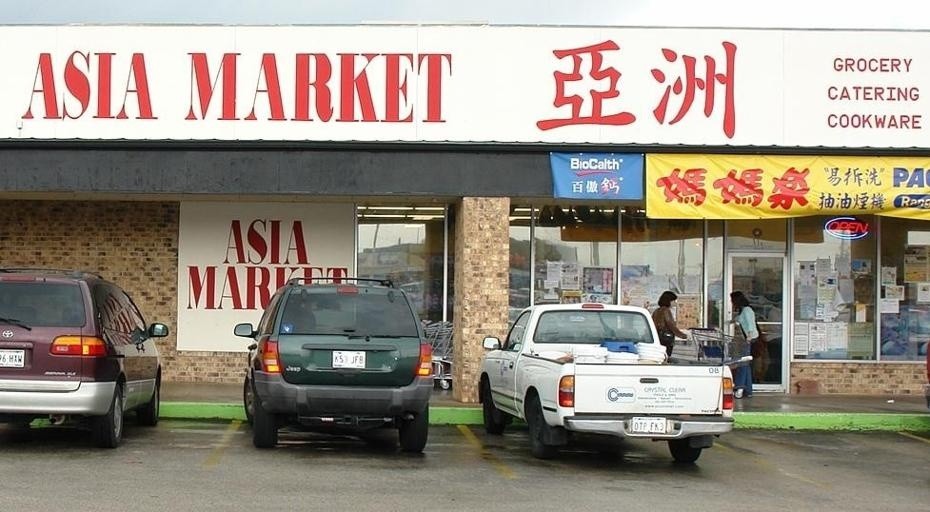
[
  {"x1": 759, "y1": 294, "x2": 783, "y2": 382},
  {"x1": 729, "y1": 290, "x2": 760, "y2": 398},
  {"x1": 651, "y1": 290, "x2": 694, "y2": 358}
]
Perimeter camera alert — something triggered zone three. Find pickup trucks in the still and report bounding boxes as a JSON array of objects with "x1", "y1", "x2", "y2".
[{"x1": 478, "y1": 303, "x2": 735, "y2": 463}]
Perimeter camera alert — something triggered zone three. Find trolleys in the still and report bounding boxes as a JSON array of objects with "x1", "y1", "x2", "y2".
[
  {"x1": 425, "y1": 321, "x2": 454, "y2": 391},
  {"x1": 689, "y1": 327, "x2": 751, "y2": 399}
]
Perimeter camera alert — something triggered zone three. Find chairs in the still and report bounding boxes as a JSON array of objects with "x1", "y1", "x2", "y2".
[
  {"x1": 13, "y1": 306, "x2": 37, "y2": 324},
  {"x1": 281, "y1": 303, "x2": 315, "y2": 332}
]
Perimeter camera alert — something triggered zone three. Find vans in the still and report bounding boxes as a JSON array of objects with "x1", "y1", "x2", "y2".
[
  {"x1": 234, "y1": 277, "x2": 433, "y2": 452},
  {"x1": 0, "y1": 265, "x2": 161, "y2": 447}
]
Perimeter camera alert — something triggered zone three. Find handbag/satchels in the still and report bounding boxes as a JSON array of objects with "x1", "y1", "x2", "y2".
[
  {"x1": 661, "y1": 329, "x2": 675, "y2": 345},
  {"x1": 748, "y1": 333, "x2": 767, "y2": 357}
]
